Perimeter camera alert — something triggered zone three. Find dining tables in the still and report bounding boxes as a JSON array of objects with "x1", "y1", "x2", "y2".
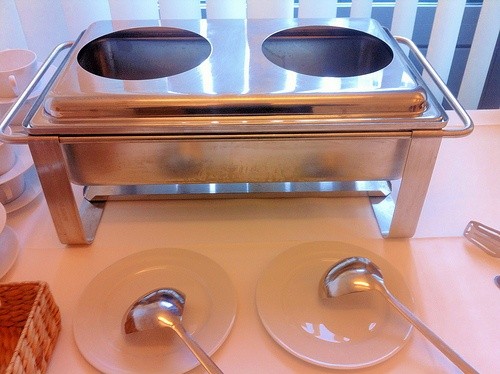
[{"x1": 0, "y1": 108, "x2": 500, "y2": 374}]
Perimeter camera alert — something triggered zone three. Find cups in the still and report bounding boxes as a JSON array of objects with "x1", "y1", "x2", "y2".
[
  {"x1": 0, "y1": 47, "x2": 38, "y2": 96},
  {"x1": 0, "y1": 173, "x2": 25, "y2": 205},
  {"x1": 0, "y1": 203, "x2": 8, "y2": 236},
  {"x1": 0, "y1": 142, "x2": 16, "y2": 175},
  {"x1": 0, "y1": 105, "x2": 37, "y2": 126}
]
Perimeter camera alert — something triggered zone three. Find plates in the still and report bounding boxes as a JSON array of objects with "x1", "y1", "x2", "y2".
[
  {"x1": 6, "y1": 124, "x2": 28, "y2": 134},
  {"x1": 0, "y1": 144, "x2": 34, "y2": 185},
  {"x1": 73, "y1": 247, "x2": 240, "y2": 374},
  {"x1": 0, "y1": 62, "x2": 60, "y2": 104},
  {"x1": 0, "y1": 224, "x2": 20, "y2": 281},
  {"x1": 3, "y1": 168, "x2": 43, "y2": 214},
  {"x1": 255, "y1": 240, "x2": 414, "y2": 369}
]
